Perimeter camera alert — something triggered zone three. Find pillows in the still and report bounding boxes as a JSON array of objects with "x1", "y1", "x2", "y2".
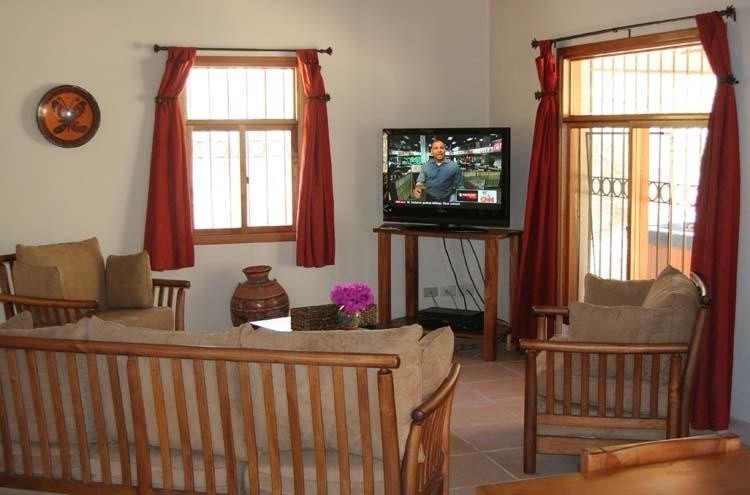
[
  {"x1": 105, "y1": 250, "x2": 155, "y2": 310},
  {"x1": 88, "y1": 315, "x2": 247, "y2": 462},
  {"x1": 12, "y1": 260, "x2": 66, "y2": 301},
  {"x1": 0, "y1": 312, "x2": 98, "y2": 447},
  {"x1": 240, "y1": 318, "x2": 424, "y2": 462},
  {"x1": 419, "y1": 325, "x2": 454, "y2": 400},
  {"x1": 15, "y1": 237, "x2": 107, "y2": 314},
  {"x1": 568, "y1": 266, "x2": 701, "y2": 386},
  {"x1": 16, "y1": 237, "x2": 107, "y2": 301}
]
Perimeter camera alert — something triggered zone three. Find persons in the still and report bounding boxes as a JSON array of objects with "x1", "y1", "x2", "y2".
[{"x1": 413, "y1": 139, "x2": 466, "y2": 203}]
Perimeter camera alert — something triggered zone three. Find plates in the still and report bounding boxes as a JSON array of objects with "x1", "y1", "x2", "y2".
[{"x1": 36, "y1": 85, "x2": 101, "y2": 149}]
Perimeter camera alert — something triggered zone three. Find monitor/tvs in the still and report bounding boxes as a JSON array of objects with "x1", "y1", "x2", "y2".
[{"x1": 383, "y1": 127, "x2": 511, "y2": 233}]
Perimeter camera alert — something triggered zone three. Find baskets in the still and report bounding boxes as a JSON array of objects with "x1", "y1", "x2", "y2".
[{"x1": 291, "y1": 302, "x2": 377, "y2": 331}]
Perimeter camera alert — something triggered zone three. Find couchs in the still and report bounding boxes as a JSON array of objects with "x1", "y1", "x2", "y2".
[{"x1": 0, "y1": 310, "x2": 461, "y2": 495}]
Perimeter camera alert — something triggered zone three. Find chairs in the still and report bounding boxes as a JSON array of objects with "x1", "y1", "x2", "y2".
[
  {"x1": 0, "y1": 237, "x2": 191, "y2": 332},
  {"x1": 579, "y1": 430, "x2": 742, "y2": 473},
  {"x1": 515, "y1": 265, "x2": 710, "y2": 473}
]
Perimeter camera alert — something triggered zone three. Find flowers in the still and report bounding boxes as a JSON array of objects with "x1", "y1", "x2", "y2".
[{"x1": 330, "y1": 283, "x2": 376, "y2": 312}]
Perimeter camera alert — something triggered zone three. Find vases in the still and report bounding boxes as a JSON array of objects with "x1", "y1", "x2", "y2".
[
  {"x1": 231, "y1": 265, "x2": 289, "y2": 326},
  {"x1": 338, "y1": 309, "x2": 361, "y2": 329}
]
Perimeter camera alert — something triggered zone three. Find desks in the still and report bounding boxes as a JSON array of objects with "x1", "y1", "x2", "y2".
[{"x1": 372, "y1": 224, "x2": 524, "y2": 361}]
